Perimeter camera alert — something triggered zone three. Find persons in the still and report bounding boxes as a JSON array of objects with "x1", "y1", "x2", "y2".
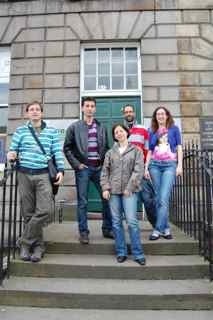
[
  {"x1": 99, "y1": 123, "x2": 147, "y2": 265},
  {"x1": 148, "y1": 106, "x2": 183, "y2": 240},
  {"x1": 62, "y1": 97, "x2": 114, "y2": 244},
  {"x1": 122, "y1": 103, "x2": 157, "y2": 229},
  {"x1": 6, "y1": 100, "x2": 65, "y2": 262}
]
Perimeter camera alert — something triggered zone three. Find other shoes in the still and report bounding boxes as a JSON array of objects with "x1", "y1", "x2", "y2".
[
  {"x1": 80, "y1": 234, "x2": 89, "y2": 244},
  {"x1": 136, "y1": 258, "x2": 145, "y2": 265},
  {"x1": 16, "y1": 239, "x2": 31, "y2": 260},
  {"x1": 149, "y1": 230, "x2": 160, "y2": 240},
  {"x1": 103, "y1": 231, "x2": 114, "y2": 239},
  {"x1": 117, "y1": 255, "x2": 127, "y2": 263},
  {"x1": 162, "y1": 228, "x2": 172, "y2": 239},
  {"x1": 30, "y1": 245, "x2": 46, "y2": 262}
]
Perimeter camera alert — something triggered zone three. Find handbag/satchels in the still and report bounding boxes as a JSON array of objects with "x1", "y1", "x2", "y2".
[{"x1": 47, "y1": 155, "x2": 59, "y2": 189}]
